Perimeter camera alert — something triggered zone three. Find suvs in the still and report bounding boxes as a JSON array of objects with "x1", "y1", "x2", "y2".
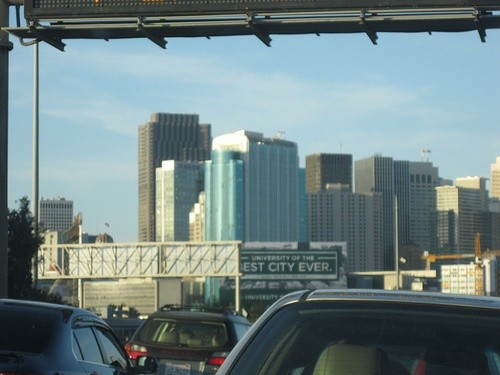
[
  {"x1": 0, "y1": 297, "x2": 160, "y2": 371},
  {"x1": 125, "y1": 302, "x2": 254, "y2": 371},
  {"x1": 213, "y1": 284, "x2": 500, "y2": 371}
]
